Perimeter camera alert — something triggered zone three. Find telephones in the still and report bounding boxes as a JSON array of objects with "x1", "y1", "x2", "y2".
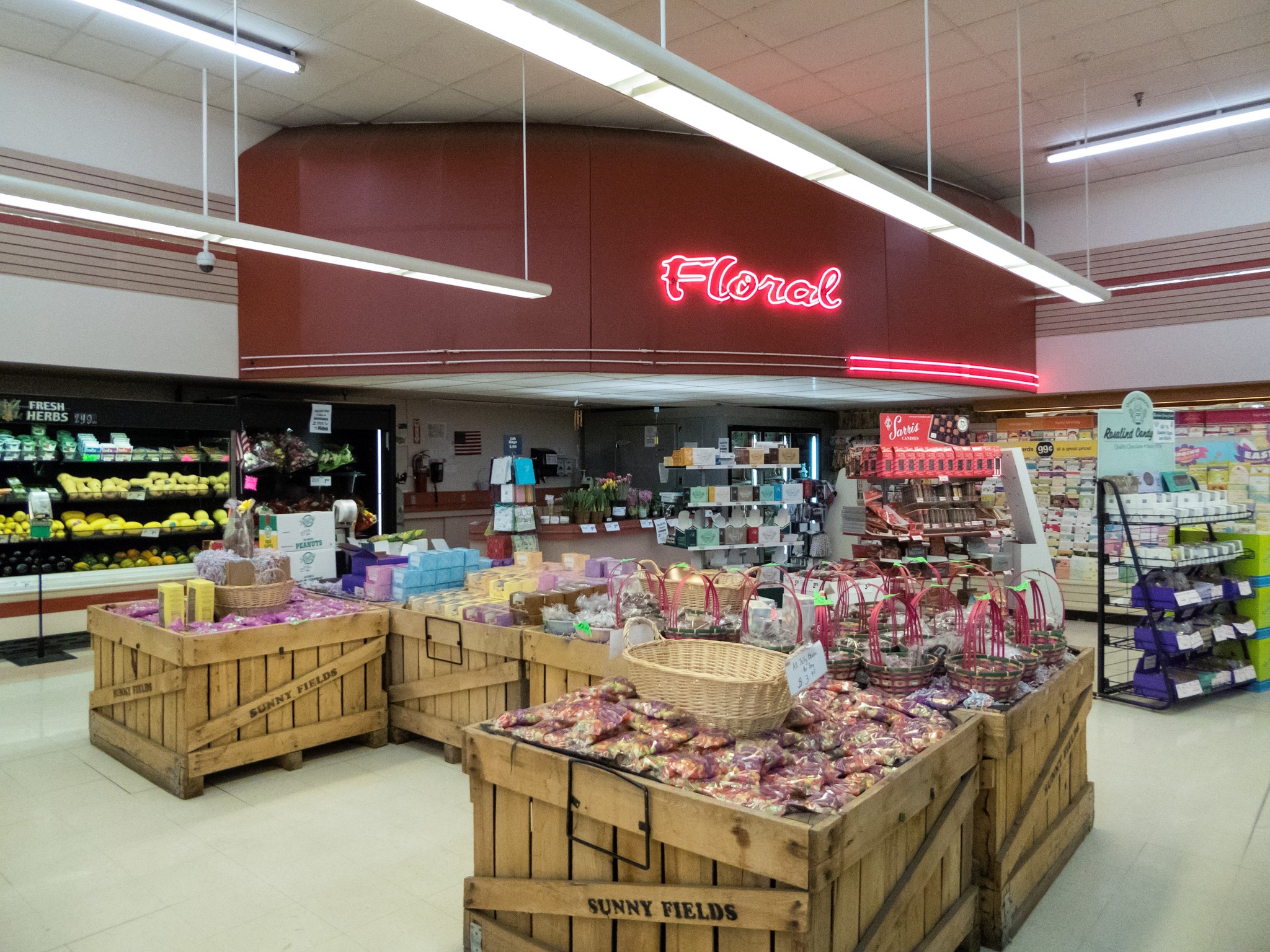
[{"x1": 431, "y1": 462, "x2": 443, "y2": 483}]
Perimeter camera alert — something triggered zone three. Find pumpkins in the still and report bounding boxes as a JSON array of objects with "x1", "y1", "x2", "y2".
[
  {"x1": 56, "y1": 472, "x2": 230, "y2": 500},
  {"x1": 71, "y1": 543, "x2": 201, "y2": 571}
]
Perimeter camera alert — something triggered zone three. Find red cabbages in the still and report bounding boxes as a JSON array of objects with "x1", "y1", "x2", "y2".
[{"x1": 0, "y1": 546, "x2": 75, "y2": 577}]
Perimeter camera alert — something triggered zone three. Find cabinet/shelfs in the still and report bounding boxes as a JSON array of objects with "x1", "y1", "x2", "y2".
[
  {"x1": 1102, "y1": 478, "x2": 1256, "y2": 706},
  {"x1": 843, "y1": 470, "x2": 1016, "y2": 577},
  {"x1": 661, "y1": 461, "x2": 804, "y2": 566}
]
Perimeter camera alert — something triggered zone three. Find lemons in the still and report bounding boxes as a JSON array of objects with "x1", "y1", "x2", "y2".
[{"x1": 0, "y1": 510, "x2": 66, "y2": 541}]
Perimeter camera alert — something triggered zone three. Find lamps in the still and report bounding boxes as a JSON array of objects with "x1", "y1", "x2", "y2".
[{"x1": 0, "y1": 0, "x2": 1270, "y2": 305}]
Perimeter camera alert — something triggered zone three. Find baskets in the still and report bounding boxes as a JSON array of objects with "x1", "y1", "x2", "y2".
[
  {"x1": 608, "y1": 554, "x2": 1068, "y2": 738},
  {"x1": 212, "y1": 568, "x2": 294, "y2": 620}
]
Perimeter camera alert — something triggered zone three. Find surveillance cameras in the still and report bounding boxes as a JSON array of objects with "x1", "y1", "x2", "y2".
[{"x1": 196, "y1": 251, "x2": 215, "y2": 273}]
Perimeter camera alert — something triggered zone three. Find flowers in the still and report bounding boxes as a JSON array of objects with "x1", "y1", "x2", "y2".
[{"x1": 600, "y1": 470, "x2": 632, "y2": 499}]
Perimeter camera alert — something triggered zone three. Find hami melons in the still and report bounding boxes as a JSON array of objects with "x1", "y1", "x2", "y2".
[{"x1": 61, "y1": 508, "x2": 228, "y2": 537}]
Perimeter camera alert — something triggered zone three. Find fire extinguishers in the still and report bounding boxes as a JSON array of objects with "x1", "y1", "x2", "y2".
[{"x1": 412, "y1": 449, "x2": 431, "y2": 493}]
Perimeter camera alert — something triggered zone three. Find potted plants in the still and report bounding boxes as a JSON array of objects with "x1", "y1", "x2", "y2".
[{"x1": 559, "y1": 488, "x2": 611, "y2": 525}]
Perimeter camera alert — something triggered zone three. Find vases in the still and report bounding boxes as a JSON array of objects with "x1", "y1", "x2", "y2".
[{"x1": 612, "y1": 499, "x2": 627, "y2": 519}]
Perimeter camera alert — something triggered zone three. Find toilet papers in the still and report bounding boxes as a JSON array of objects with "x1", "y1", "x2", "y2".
[{"x1": 332, "y1": 499, "x2": 358, "y2": 523}]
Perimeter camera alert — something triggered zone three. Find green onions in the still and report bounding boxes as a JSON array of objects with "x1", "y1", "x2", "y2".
[{"x1": 560, "y1": 485, "x2": 607, "y2": 513}]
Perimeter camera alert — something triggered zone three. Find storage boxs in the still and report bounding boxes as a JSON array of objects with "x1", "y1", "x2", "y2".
[
  {"x1": 841, "y1": 441, "x2": 1013, "y2": 580},
  {"x1": 342, "y1": 529, "x2": 639, "y2": 627},
  {"x1": 1105, "y1": 486, "x2": 1253, "y2": 692},
  {"x1": 186, "y1": 578, "x2": 215, "y2": 625},
  {"x1": 258, "y1": 508, "x2": 338, "y2": 552},
  {"x1": 659, "y1": 447, "x2": 803, "y2": 547},
  {"x1": 289, "y1": 548, "x2": 336, "y2": 582},
  {"x1": 156, "y1": 579, "x2": 184, "y2": 629}
]
